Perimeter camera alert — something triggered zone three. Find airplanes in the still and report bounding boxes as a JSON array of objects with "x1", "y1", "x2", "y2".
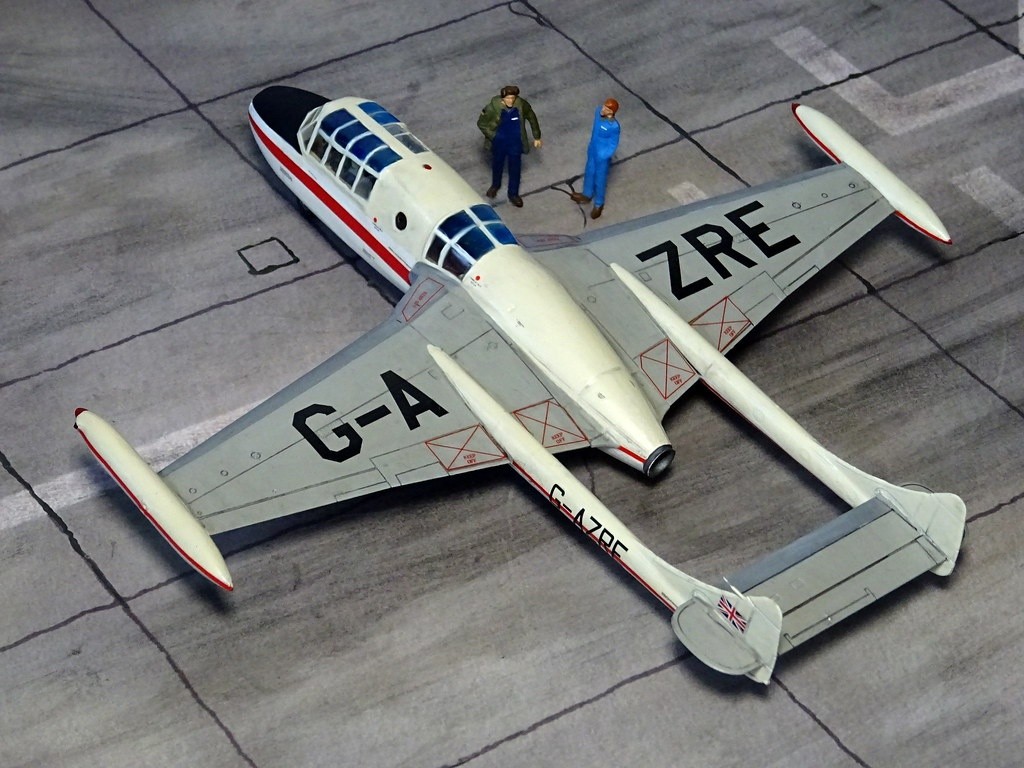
[{"x1": 72, "y1": 84, "x2": 967, "y2": 685}]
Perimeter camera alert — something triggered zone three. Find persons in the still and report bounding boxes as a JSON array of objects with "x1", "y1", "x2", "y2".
[
  {"x1": 571, "y1": 97, "x2": 621, "y2": 218},
  {"x1": 476, "y1": 86, "x2": 542, "y2": 206}
]
